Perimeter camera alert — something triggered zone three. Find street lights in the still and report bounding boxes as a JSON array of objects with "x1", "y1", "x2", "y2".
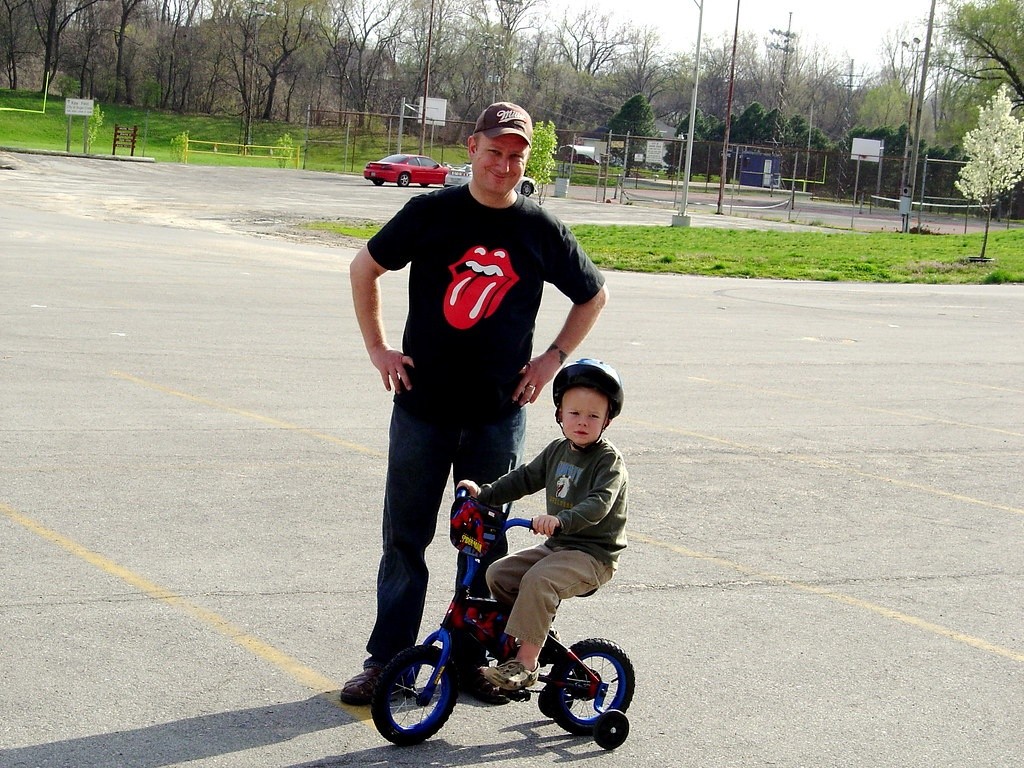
[
  {"x1": 768, "y1": 12, "x2": 797, "y2": 147},
  {"x1": 899, "y1": 38, "x2": 934, "y2": 200},
  {"x1": 244, "y1": 0, "x2": 277, "y2": 150},
  {"x1": 475, "y1": 31, "x2": 506, "y2": 105}
]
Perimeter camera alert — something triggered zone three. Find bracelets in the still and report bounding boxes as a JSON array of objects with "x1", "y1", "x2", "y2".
[{"x1": 547, "y1": 343, "x2": 568, "y2": 365}]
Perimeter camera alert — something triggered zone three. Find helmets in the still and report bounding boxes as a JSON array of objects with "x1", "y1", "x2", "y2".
[{"x1": 552, "y1": 357, "x2": 624, "y2": 419}]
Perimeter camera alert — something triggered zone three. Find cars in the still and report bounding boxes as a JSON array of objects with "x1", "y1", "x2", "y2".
[
  {"x1": 443, "y1": 161, "x2": 536, "y2": 197},
  {"x1": 364, "y1": 154, "x2": 450, "y2": 188},
  {"x1": 568, "y1": 153, "x2": 600, "y2": 166}
]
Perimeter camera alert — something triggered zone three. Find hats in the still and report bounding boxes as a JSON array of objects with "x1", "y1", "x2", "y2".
[{"x1": 473, "y1": 101, "x2": 534, "y2": 148}]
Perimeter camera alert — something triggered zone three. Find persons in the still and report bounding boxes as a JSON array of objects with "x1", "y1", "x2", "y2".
[
  {"x1": 339, "y1": 101, "x2": 608, "y2": 709},
  {"x1": 455, "y1": 358, "x2": 628, "y2": 689}
]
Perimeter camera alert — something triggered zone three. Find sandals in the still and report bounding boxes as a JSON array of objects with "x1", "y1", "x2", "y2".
[
  {"x1": 484, "y1": 659, "x2": 541, "y2": 691},
  {"x1": 514, "y1": 629, "x2": 562, "y2": 652}
]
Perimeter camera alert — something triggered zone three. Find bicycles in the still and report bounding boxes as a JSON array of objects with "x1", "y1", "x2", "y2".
[{"x1": 371, "y1": 487, "x2": 636, "y2": 751}]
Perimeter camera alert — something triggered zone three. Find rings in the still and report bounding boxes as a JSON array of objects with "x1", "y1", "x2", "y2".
[{"x1": 528, "y1": 384, "x2": 534, "y2": 388}]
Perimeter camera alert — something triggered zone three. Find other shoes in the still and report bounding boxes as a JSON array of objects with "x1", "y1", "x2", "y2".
[
  {"x1": 458, "y1": 674, "x2": 510, "y2": 705},
  {"x1": 341, "y1": 666, "x2": 411, "y2": 706}
]
